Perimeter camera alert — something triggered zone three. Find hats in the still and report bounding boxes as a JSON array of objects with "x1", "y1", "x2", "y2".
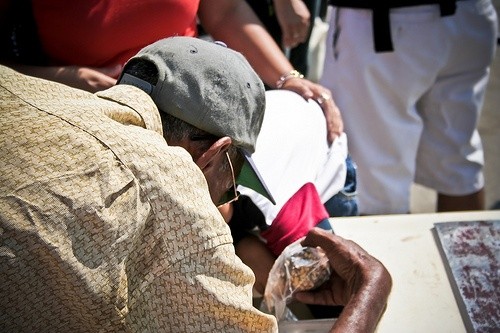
[{"x1": 112, "y1": 35, "x2": 266, "y2": 159}]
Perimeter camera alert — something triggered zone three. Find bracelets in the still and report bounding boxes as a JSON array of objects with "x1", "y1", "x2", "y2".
[{"x1": 276, "y1": 70, "x2": 304, "y2": 89}]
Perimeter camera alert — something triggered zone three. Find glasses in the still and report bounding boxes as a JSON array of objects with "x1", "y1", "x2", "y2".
[{"x1": 190, "y1": 133, "x2": 241, "y2": 208}]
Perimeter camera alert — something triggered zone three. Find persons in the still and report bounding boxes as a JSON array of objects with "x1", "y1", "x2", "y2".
[
  {"x1": 320, "y1": 0, "x2": 500, "y2": 217},
  {"x1": 0, "y1": 1, "x2": 393, "y2": 333}
]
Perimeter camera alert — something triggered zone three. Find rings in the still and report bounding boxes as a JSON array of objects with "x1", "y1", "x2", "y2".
[{"x1": 291, "y1": 33, "x2": 300, "y2": 39}]
